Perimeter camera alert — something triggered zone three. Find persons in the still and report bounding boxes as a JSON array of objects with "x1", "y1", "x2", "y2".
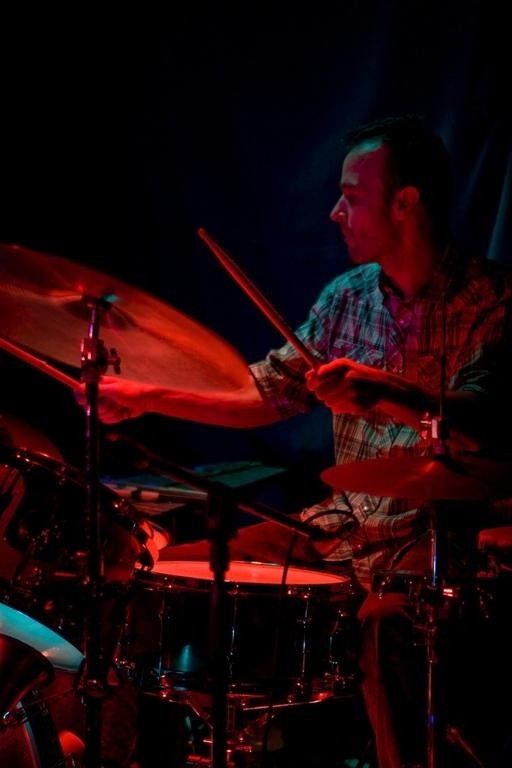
[{"x1": 74, "y1": 121, "x2": 511, "y2": 768}]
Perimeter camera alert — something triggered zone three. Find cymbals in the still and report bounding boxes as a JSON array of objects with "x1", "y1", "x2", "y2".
[
  {"x1": 0, "y1": 244, "x2": 249, "y2": 392},
  {"x1": 319, "y1": 451, "x2": 512, "y2": 502}
]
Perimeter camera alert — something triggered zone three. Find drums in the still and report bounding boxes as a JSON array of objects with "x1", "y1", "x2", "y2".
[
  {"x1": 2, "y1": 666, "x2": 197, "y2": 768},
  {"x1": 121, "y1": 558, "x2": 353, "y2": 706},
  {"x1": 0, "y1": 448, "x2": 165, "y2": 642}
]
